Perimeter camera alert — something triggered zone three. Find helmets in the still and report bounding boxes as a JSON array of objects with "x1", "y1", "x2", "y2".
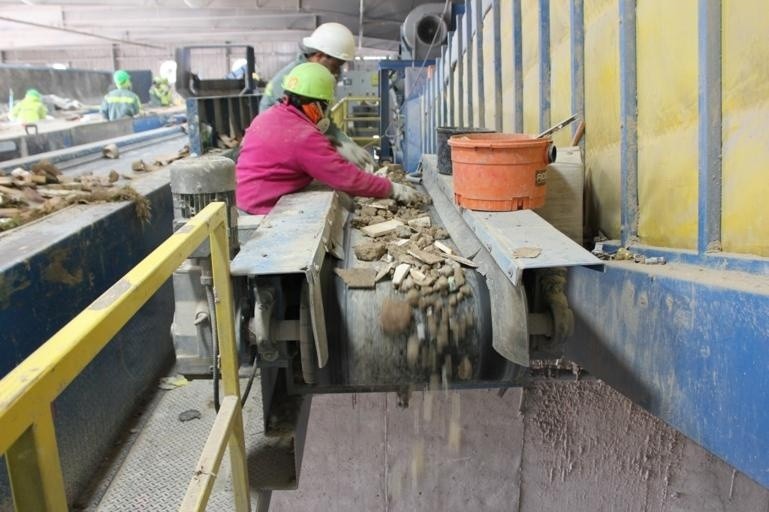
[
  {"x1": 282, "y1": 62, "x2": 334, "y2": 101},
  {"x1": 113, "y1": 71, "x2": 128, "y2": 86},
  {"x1": 302, "y1": 22, "x2": 356, "y2": 62},
  {"x1": 25, "y1": 89, "x2": 41, "y2": 99}
]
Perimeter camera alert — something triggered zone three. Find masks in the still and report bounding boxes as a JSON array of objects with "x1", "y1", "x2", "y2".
[{"x1": 302, "y1": 103, "x2": 330, "y2": 135}]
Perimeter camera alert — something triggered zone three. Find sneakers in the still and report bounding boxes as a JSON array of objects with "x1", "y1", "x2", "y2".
[{"x1": 265, "y1": 410, "x2": 296, "y2": 431}]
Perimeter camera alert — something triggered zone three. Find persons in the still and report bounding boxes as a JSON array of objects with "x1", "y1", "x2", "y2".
[
  {"x1": 101, "y1": 70, "x2": 142, "y2": 121},
  {"x1": 8, "y1": 89, "x2": 47, "y2": 124},
  {"x1": 160, "y1": 77, "x2": 173, "y2": 105},
  {"x1": 235, "y1": 62, "x2": 409, "y2": 216},
  {"x1": 148, "y1": 76, "x2": 170, "y2": 105},
  {"x1": 256, "y1": 21, "x2": 372, "y2": 170}
]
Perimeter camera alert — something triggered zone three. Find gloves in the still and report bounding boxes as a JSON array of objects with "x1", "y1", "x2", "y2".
[{"x1": 388, "y1": 182, "x2": 423, "y2": 208}]
